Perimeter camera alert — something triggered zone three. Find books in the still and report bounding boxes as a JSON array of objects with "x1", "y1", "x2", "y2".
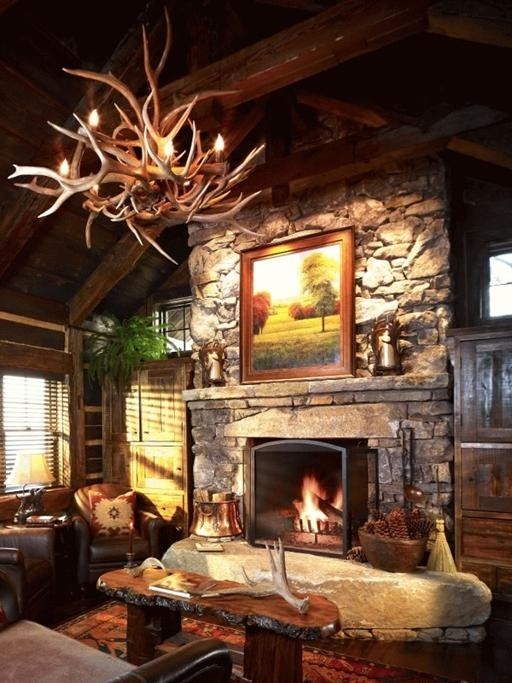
[
  {"x1": 149, "y1": 569, "x2": 218, "y2": 600},
  {"x1": 25, "y1": 520, "x2": 54, "y2": 527},
  {"x1": 26, "y1": 515, "x2": 55, "y2": 523}
]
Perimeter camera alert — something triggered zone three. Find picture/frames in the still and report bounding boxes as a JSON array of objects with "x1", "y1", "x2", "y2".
[{"x1": 239, "y1": 226, "x2": 355, "y2": 385}]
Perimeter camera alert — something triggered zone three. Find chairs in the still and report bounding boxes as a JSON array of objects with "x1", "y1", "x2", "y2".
[
  {"x1": 1, "y1": 527, "x2": 61, "y2": 628},
  {"x1": 68, "y1": 484, "x2": 176, "y2": 597}
]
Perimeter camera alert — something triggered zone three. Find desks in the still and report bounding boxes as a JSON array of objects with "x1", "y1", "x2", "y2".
[{"x1": 2, "y1": 517, "x2": 72, "y2": 573}]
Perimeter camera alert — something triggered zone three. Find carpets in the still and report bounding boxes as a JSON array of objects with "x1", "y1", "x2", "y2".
[{"x1": 53, "y1": 602, "x2": 470, "y2": 682}]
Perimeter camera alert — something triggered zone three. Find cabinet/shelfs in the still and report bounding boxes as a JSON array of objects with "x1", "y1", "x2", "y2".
[
  {"x1": 101, "y1": 350, "x2": 195, "y2": 538},
  {"x1": 446, "y1": 322, "x2": 512, "y2": 624}
]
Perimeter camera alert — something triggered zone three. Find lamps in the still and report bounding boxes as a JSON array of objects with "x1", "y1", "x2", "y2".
[
  {"x1": 359, "y1": 309, "x2": 407, "y2": 376},
  {"x1": 198, "y1": 338, "x2": 237, "y2": 384},
  {"x1": 8, "y1": 0, "x2": 266, "y2": 266}
]
